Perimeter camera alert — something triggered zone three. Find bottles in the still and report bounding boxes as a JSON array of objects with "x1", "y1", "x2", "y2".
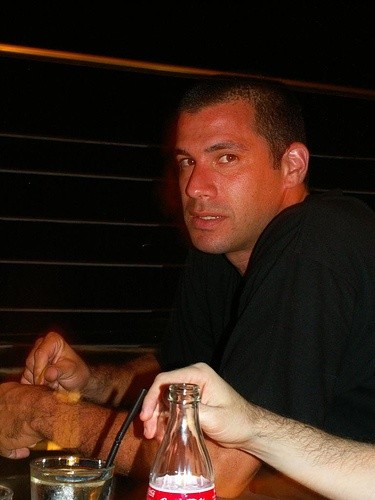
[{"x1": 146, "y1": 383, "x2": 217, "y2": 500}]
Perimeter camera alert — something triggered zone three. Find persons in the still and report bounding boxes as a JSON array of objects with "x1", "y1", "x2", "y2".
[
  {"x1": 137, "y1": 358, "x2": 374, "y2": 500},
  {"x1": 1, "y1": 70, "x2": 374, "y2": 499}
]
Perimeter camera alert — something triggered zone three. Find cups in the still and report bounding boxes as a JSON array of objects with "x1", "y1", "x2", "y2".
[{"x1": 30, "y1": 455, "x2": 116, "y2": 500}]
